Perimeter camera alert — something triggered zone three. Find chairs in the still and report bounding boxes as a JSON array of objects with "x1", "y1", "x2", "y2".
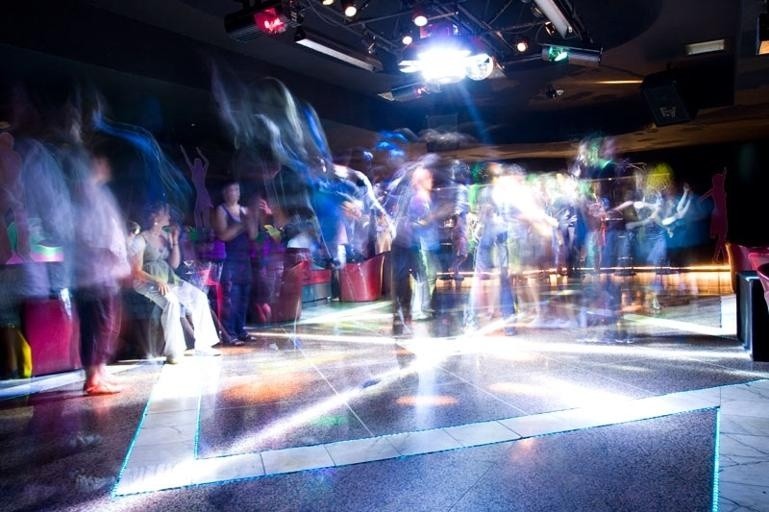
[
  {"x1": 339, "y1": 253, "x2": 386, "y2": 302},
  {"x1": 725, "y1": 242, "x2": 769, "y2": 314},
  {"x1": 249, "y1": 259, "x2": 305, "y2": 323}
]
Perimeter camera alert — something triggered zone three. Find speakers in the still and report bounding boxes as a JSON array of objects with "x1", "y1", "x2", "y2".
[{"x1": 735, "y1": 269, "x2": 769, "y2": 362}]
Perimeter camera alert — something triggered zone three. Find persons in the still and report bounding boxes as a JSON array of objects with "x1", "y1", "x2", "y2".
[{"x1": 0, "y1": 87, "x2": 697, "y2": 398}]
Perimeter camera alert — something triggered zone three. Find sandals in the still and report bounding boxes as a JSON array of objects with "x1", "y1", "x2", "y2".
[{"x1": 83, "y1": 374, "x2": 122, "y2": 394}]
